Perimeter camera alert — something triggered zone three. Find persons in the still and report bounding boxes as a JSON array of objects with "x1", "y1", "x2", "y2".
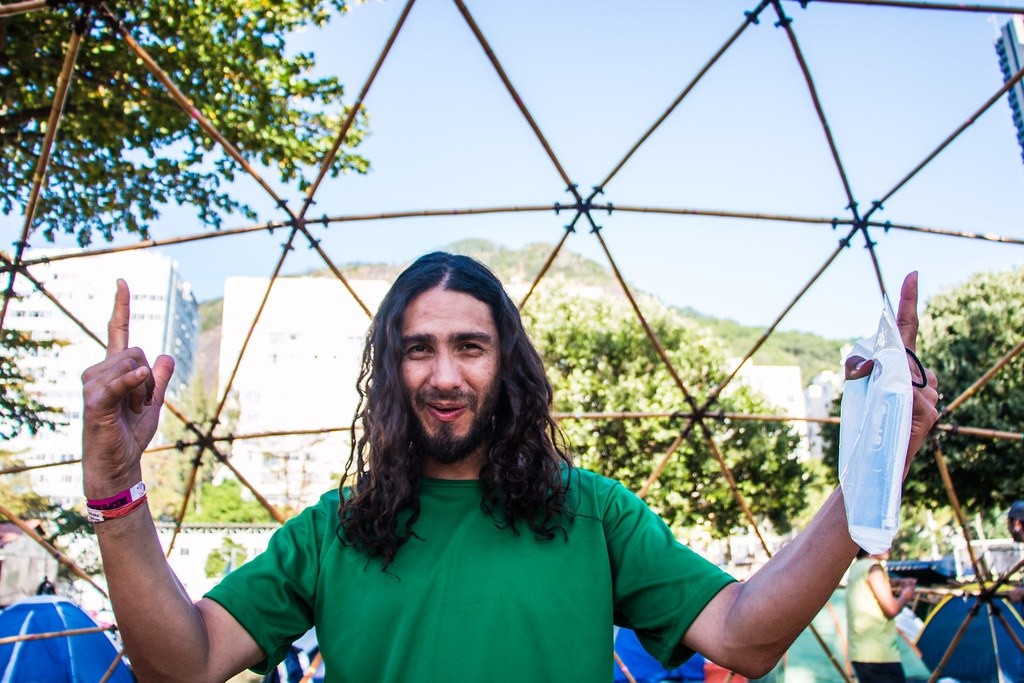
[
  {"x1": 81, "y1": 251, "x2": 939, "y2": 683},
  {"x1": 845, "y1": 546, "x2": 918, "y2": 683},
  {"x1": 1007, "y1": 502, "x2": 1024, "y2": 604},
  {"x1": 36, "y1": 576, "x2": 56, "y2": 596}
]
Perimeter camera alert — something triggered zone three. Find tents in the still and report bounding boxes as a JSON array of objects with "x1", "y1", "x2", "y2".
[
  {"x1": 0, "y1": 595, "x2": 133, "y2": 683},
  {"x1": 916, "y1": 582, "x2": 1024, "y2": 683}
]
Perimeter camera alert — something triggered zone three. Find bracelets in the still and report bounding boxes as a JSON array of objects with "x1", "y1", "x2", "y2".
[
  {"x1": 85, "y1": 481, "x2": 148, "y2": 522},
  {"x1": 896, "y1": 579, "x2": 900, "y2": 586}
]
